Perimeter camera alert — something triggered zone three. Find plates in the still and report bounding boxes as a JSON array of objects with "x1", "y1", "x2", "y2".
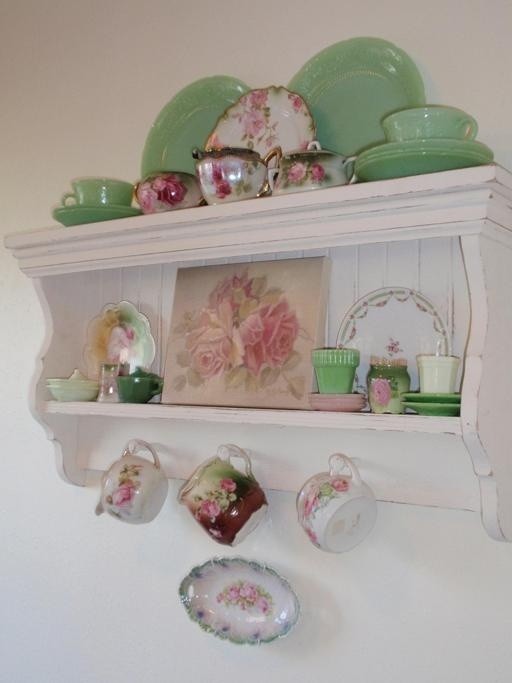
[
  {"x1": 202, "y1": 85, "x2": 318, "y2": 199},
  {"x1": 138, "y1": 75, "x2": 254, "y2": 180},
  {"x1": 82, "y1": 301, "x2": 158, "y2": 376},
  {"x1": 178, "y1": 555, "x2": 301, "y2": 646},
  {"x1": 354, "y1": 140, "x2": 493, "y2": 182},
  {"x1": 400, "y1": 392, "x2": 460, "y2": 417},
  {"x1": 53, "y1": 205, "x2": 144, "y2": 227},
  {"x1": 309, "y1": 392, "x2": 366, "y2": 411},
  {"x1": 285, "y1": 36, "x2": 429, "y2": 158},
  {"x1": 335, "y1": 286, "x2": 455, "y2": 403}
]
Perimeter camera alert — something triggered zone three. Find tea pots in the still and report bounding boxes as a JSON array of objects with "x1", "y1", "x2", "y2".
[{"x1": 94, "y1": 438, "x2": 170, "y2": 525}]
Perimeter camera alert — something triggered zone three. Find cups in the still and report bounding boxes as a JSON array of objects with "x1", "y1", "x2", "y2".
[
  {"x1": 116, "y1": 370, "x2": 163, "y2": 404},
  {"x1": 365, "y1": 365, "x2": 411, "y2": 415},
  {"x1": 379, "y1": 103, "x2": 480, "y2": 145},
  {"x1": 312, "y1": 349, "x2": 359, "y2": 394},
  {"x1": 416, "y1": 354, "x2": 460, "y2": 394},
  {"x1": 176, "y1": 442, "x2": 269, "y2": 547},
  {"x1": 276, "y1": 141, "x2": 355, "y2": 195},
  {"x1": 296, "y1": 452, "x2": 379, "y2": 555},
  {"x1": 192, "y1": 146, "x2": 282, "y2": 207},
  {"x1": 96, "y1": 361, "x2": 121, "y2": 403},
  {"x1": 62, "y1": 177, "x2": 134, "y2": 205},
  {"x1": 133, "y1": 170, "x2": 202, "y2": 213}
]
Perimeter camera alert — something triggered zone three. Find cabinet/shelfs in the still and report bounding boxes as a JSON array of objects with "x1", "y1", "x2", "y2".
[{"x1": 4, "y1": 162, "x2": 510, "y2": 547}]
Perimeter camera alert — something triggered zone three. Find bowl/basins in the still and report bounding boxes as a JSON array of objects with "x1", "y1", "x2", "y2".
[{"x1": 46, "y1": 378, "x2": 98, "y2": 401}]
